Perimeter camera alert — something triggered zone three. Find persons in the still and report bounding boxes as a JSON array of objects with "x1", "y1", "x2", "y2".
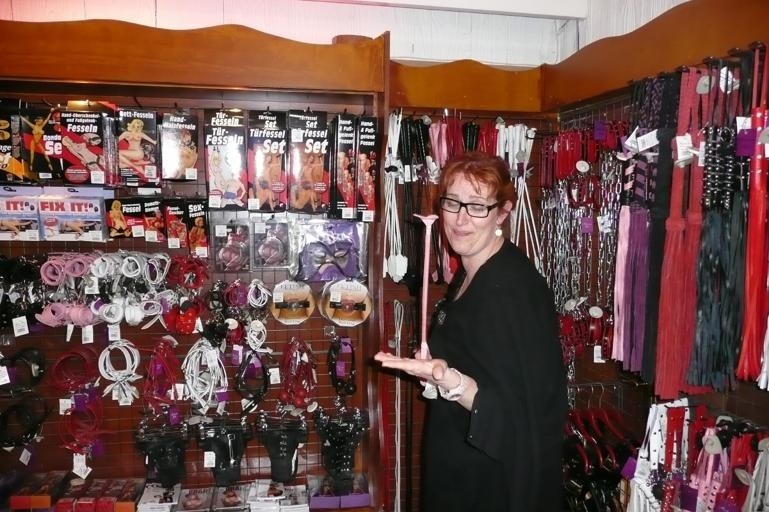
[{"x1": 370, "y1": 145, "x2": 574, "y2": 510}]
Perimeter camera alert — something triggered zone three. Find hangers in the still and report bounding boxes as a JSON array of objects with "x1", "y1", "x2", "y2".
[{"x1": 565, "y1": 381, "x2": 644, "y2": 475}]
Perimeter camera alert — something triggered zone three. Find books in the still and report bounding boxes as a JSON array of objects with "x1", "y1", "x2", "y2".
[{"x1": 136, "y1": 479, "x2": 311, "y2": 512}]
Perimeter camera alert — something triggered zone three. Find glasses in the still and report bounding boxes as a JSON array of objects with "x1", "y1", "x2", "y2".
[{"x1": 439, "y1": 196, "x2": 500, "y2": 218}]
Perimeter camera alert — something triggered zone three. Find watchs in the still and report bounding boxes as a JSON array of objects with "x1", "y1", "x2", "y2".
[{"x1": 435, "y1": 366, "x2": 468, "y2": 401}]
